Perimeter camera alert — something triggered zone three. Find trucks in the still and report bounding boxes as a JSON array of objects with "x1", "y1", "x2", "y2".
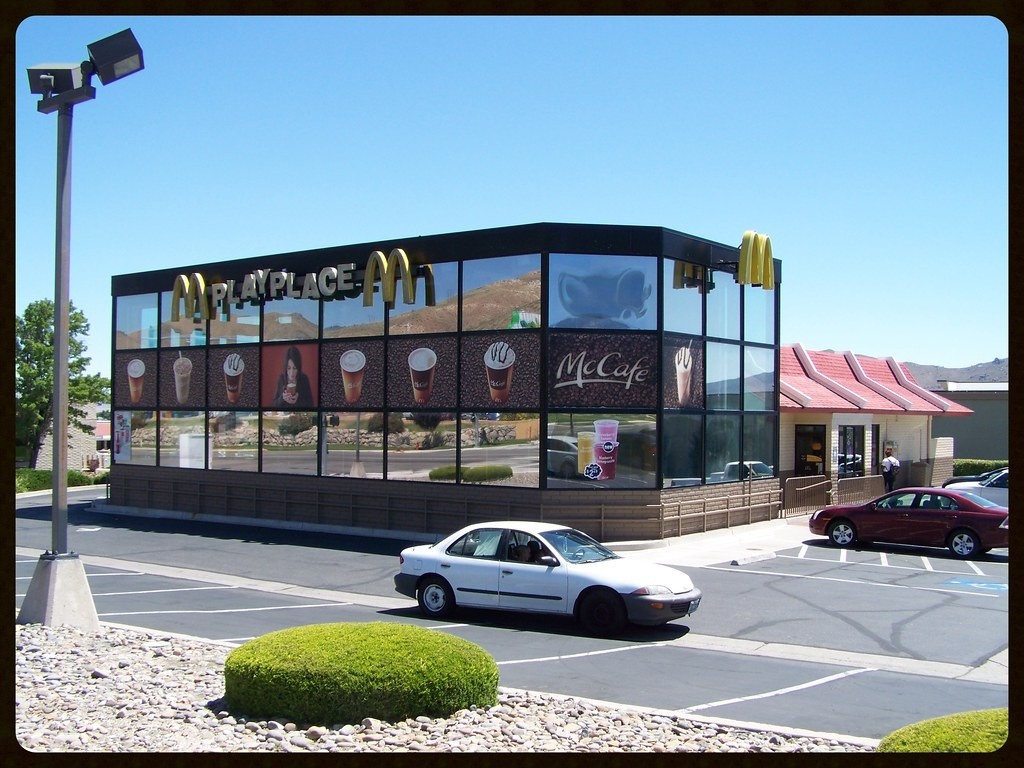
[{"x1": 670, "y1": 461, "x2": 773, "y2": 489}]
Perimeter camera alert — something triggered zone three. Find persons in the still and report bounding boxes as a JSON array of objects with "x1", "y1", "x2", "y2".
[
  {"x1": 882, "y1": 450, "x2": 900, "y2": 493},
  {"x1": 272, "y1": 348, "x2": 314, "y2": 409},
  {"x1": 512, "y1": 540, "x2": 558, "y2": 563}
]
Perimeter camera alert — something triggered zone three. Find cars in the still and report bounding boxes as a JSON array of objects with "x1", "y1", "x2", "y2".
[
  {"x1": 942, "y1": 467, "x2": 1009, "y2": 489},
  {"x1": 943, "y1": 469, "x2": 1010, "y2": 507},
  {"x1": 393, "y1": 521, "x2": 705, "y2": 636},
  {"x1": 809, "y1": 486, "x2": 1010, "y2": 560},
  {"x1": 837, "y1": 454, "x2": 864, "y2": 478},
  {"x1": 485, "y1": 413, "x2": 501, "y2": 420},
  {"x1": 547, "y1": 435, "x2": 588, "y2": 479}
]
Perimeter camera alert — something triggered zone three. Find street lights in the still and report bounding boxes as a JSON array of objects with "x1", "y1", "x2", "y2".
[{"x1": 26, "y1": 28, "x2": 147, "y2": 561}]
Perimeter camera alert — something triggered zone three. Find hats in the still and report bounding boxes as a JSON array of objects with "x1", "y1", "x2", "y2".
[{"x1": 885, "y1": 448, "x2": 892, "y2": 454}]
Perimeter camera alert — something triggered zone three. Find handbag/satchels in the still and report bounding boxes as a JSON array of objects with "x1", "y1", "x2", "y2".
[{"x1": 891, "y1": 465, "x2": 899, "y2": 476}]
